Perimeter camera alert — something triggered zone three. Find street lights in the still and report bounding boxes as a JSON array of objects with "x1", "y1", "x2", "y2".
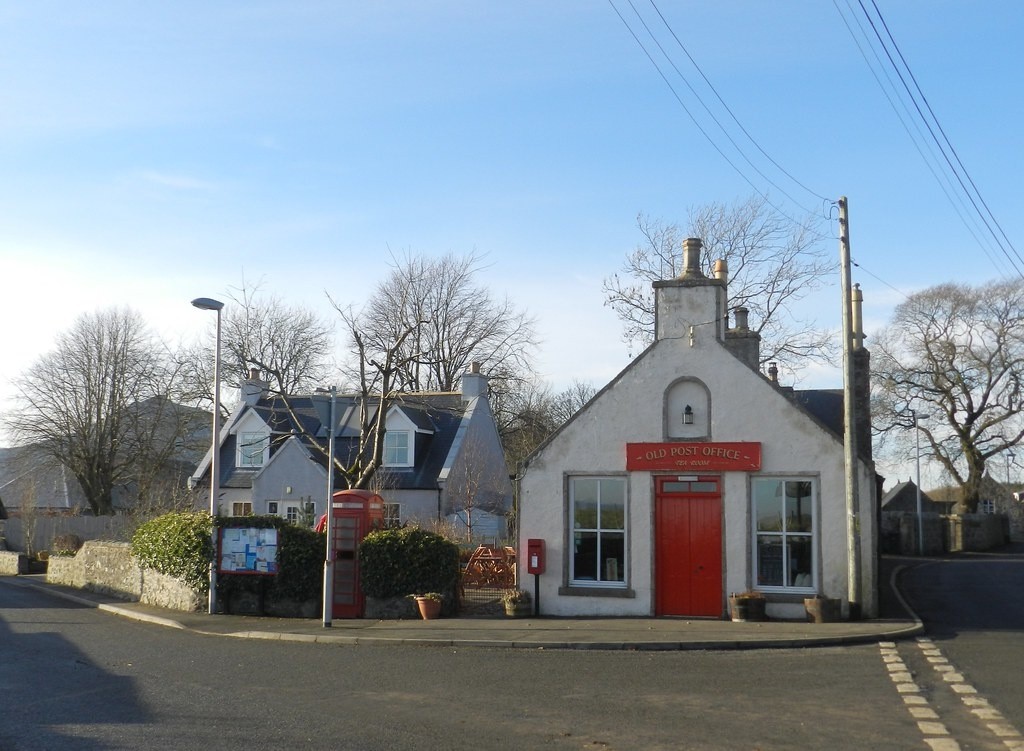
[
  {"x1": 191, "y1": 297, "x2": 225, "y2": 612},
  {"x1": 314, "y1": 388, "x2": 337, "y2": 628},
  {"x1": 914, "y1": 414, "x2": 931, "y2": 556},
  {"x1": 1006, "y1": 452, "x2": 1016, "y2": 547}
]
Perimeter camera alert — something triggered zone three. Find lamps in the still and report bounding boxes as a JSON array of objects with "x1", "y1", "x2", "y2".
[{"x1": 683, "y1": 404, "x2": 694, "y2": 424}]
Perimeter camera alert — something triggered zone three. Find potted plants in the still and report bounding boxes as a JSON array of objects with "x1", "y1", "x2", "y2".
[
  {"x1": 805, "y1": 594, "x2": 841, "y2": 623},
  {"x1": 415, "y1": 592, "x2": 444, "y2": 619},
  {"x1": 729, "y1": 591, "x2": 766, "y2": 623},
  {"x1": 505, "y1": 591, "x2": 530, "y2": 618}
]
